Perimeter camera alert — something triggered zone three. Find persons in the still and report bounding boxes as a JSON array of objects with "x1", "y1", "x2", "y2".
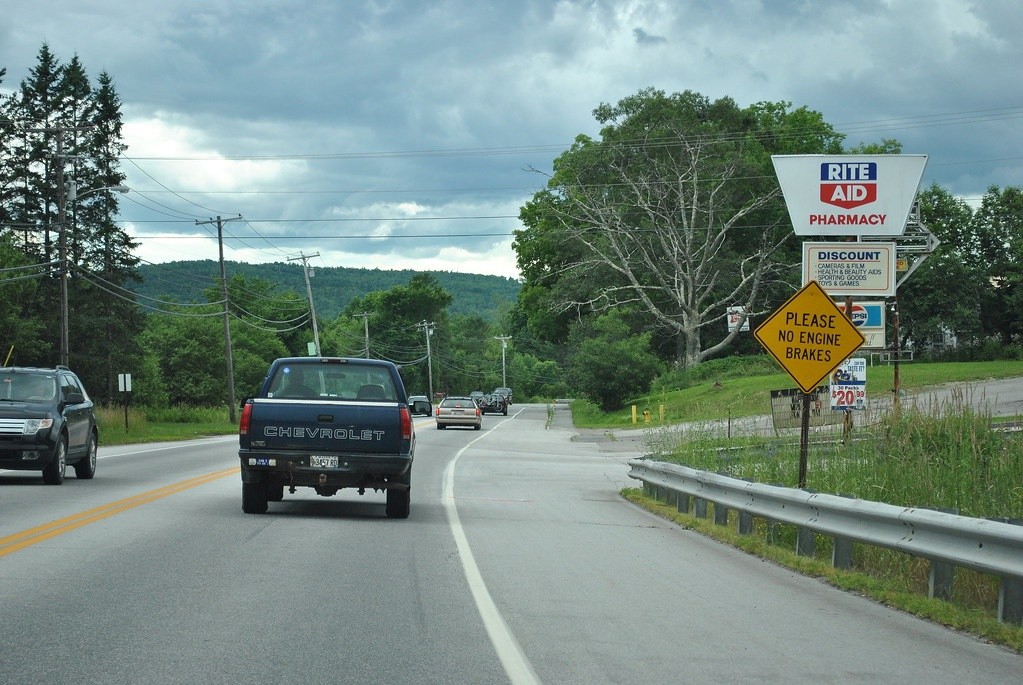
[
  {"x1": 270, "y1": 367, "x2": 320, "y2": 398},
  {"x1": 36, "y1": 382, "x2": 52, "y2": 399},
  {"x1": 841, "y1": 370, "x2": 850, "y2": 380}
]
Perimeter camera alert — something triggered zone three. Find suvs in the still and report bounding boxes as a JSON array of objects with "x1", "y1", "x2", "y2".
[{"x1": 0, "y1": 365, "x2": 100, "y2": 486}]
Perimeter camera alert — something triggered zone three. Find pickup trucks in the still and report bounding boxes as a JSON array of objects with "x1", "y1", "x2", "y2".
[{"x1": 238, "y1": 356, "x2": 432, "y2": 519}]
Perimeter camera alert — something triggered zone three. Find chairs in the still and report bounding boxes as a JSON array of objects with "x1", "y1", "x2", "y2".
[
  {"x1": 357, "y1": 384, "x2": 387, "y2": 401},
  {"x1": 285, "y1": 383, "x2": 313, "y2": 398}
]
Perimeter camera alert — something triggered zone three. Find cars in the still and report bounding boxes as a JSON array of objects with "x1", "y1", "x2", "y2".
[
  {"x1": 478, "y1": 394, "x2": 508, "y2": 416},
  {"x1": 470, "y1": 391, "x2": 483, "y2": 401},
  {"x1": 408, "y1": 396, "x2": 432, "y2": 417},
  {"x1": 436, "y1": 396, "x2": 482, "y2": 430},
  {"x1": 491, "y1": 387, "x2": 514, "y2": 406}
]
